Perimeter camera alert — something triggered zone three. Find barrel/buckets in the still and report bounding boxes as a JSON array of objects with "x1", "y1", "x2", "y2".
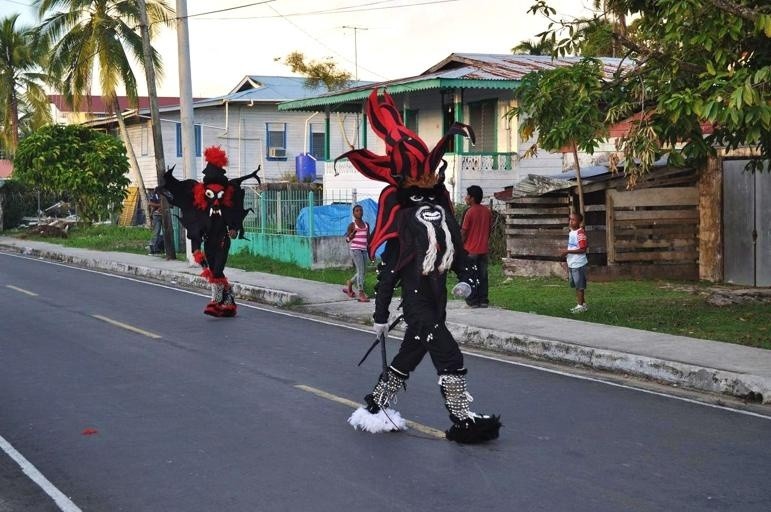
[{"x1": 296, "y1": 154, "x2": 317, "y2": 183}]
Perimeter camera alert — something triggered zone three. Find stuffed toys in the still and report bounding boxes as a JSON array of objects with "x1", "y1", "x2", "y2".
[
  {"x1": 332, "y1": 86, "x2": 503, "y2": 447},
  {"x1": 156, "y1": 145, "x2": 260, "y2": 317}
]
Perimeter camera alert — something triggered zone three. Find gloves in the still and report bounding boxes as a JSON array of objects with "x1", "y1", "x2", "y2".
[
  {"x1": 373, "y1": 323, "x2": 388, "y2": 341},
  {"x1": 451, "y1": 282, "x2": 472, "y2": 298}
]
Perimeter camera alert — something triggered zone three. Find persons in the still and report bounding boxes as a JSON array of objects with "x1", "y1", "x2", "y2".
[
  {"x1": 341, "y1": 205, "x2": 370, "y2": 303},
  {"x1": 458, "y1": 192, "x2": 472, "y2": 229},
  {"x1": 458, "y1": 184, "x2": 495, "y2": 308},
  {"x1": 557, "y1": 212, "x2": 589, "y2": 314}
]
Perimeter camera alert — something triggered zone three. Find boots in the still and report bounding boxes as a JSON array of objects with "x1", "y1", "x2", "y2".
[
  {"x1": 203, "y1": 277, "x2": 237, "y2": 317},
  {"x1": 438, "y1": 368, "x2": 500, "y2": 443},
  {"x1": 348, "y1": 366, "x2": 409, "y2": 433}
]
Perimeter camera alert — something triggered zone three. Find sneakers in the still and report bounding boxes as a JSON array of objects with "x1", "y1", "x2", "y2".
[
  {"x1": 570, "y1": 303, "x2": 588, "y2": 313},
  {"x1": 470, "y1": 303, "x2": 487, "y2": 308}
]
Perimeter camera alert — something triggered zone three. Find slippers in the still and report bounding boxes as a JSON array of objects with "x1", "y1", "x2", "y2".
[
  {"x1": 357, "y1": 295, "x2": 369, "y2": 301},
  {"x1": 343, "y1": 288, "x2": 355, "y2": 296}
]
